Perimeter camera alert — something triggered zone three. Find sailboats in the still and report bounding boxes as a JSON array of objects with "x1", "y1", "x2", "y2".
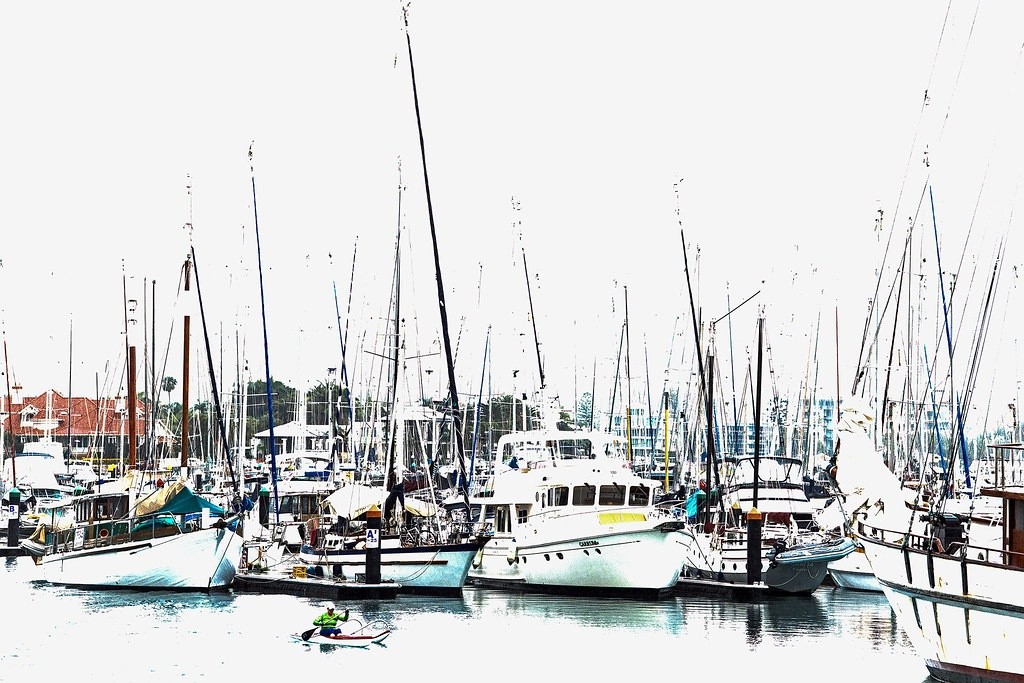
[{"x1": 0, "y1": 0, "x2": 1024, "y2": 683}]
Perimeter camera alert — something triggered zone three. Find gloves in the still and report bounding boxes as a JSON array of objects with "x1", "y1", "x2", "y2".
[
  {"x1": 345, "y1": 608, "x2": 349, "y2": 614},
  {"x1": 320, "y1": 622, "x2": 325, "y2": 626}
]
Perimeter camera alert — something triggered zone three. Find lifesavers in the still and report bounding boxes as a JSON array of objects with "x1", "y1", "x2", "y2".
[{"x1": 99, "y1": 529, "x2": 110, "y2": 538}]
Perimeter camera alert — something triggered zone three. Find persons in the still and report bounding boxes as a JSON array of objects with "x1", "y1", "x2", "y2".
[
  {"x1": 157, "y1": 478, "x2": 165, "y2": 489},
  {"x1": 313, "y1": 604, "x2": 349, "y2": 637}
]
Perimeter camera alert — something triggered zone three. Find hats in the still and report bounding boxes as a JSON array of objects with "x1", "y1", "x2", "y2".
[{"x1": 326, "y1": 602, "x2": 336, "y2": 611}]
